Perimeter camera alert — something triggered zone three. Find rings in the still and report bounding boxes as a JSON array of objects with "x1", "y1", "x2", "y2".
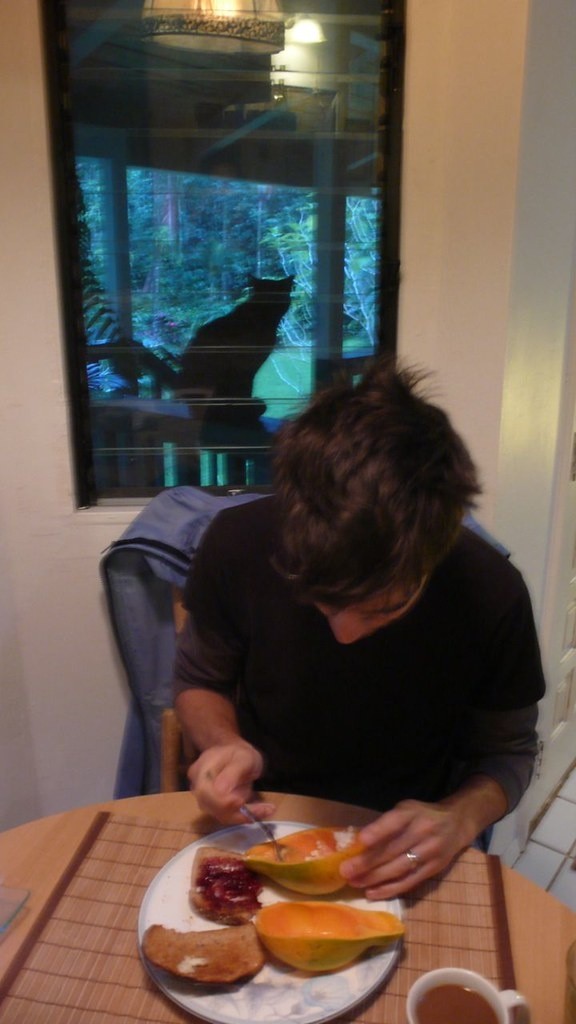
[{"x1": 405, "y1": 850, "x2": 420, "y2": 869}]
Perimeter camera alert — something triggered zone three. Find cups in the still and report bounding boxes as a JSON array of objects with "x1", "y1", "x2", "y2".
[{"x1": 405, "y1": 967, "x2": 530, "y2": 1024}]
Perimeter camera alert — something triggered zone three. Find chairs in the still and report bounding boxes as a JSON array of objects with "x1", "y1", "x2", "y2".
[{"x1": 91, "y1": 484, "x2": 511, "y2": 795}]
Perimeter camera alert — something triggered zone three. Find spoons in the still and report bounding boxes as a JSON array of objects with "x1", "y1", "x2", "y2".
[{"x1": 208, "y1": 773, "x2": 309, "y2": 862}]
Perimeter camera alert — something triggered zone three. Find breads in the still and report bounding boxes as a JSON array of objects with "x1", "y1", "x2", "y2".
[
  {"x1": 142, "y1": 921, "x2": 265, "y2": 993},
  {"x1": 189, "y1": 846, "x2": 262, "y2": 926}
]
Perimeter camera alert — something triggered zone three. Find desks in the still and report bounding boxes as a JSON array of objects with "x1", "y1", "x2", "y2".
[{"x1": 0, "y1": 792, "x2": 576, "y2": 1024}]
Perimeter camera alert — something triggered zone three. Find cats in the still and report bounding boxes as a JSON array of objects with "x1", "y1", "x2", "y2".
[{"x1": 177, "y1": 273, "x2": 296, "y2": 421}]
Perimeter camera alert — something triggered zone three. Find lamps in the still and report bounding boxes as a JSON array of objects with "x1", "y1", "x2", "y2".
[
  {"x1": 287, "y1": 13, "x2": 327, "y2": 43},
  {"x1": 137, "y1": 0, "x2": 285, "y2": 56}
]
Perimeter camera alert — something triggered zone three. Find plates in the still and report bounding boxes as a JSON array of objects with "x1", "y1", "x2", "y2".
[{"x1": 139, "y1": 820, "x2": 405, "y2": 1023}]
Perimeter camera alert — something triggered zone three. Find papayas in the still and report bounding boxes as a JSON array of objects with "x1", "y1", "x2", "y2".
[
  {"x1": 243, "y1": 826, "x2": 360, "y2": 894},
  {"x1": 255, "y1": 902, "x2": 404, "y2": 972}
]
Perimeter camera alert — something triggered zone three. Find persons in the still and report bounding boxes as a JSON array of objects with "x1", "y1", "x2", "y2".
[{"x1": 169, "y1": 351, "x2": 546, "y2": 901}]
